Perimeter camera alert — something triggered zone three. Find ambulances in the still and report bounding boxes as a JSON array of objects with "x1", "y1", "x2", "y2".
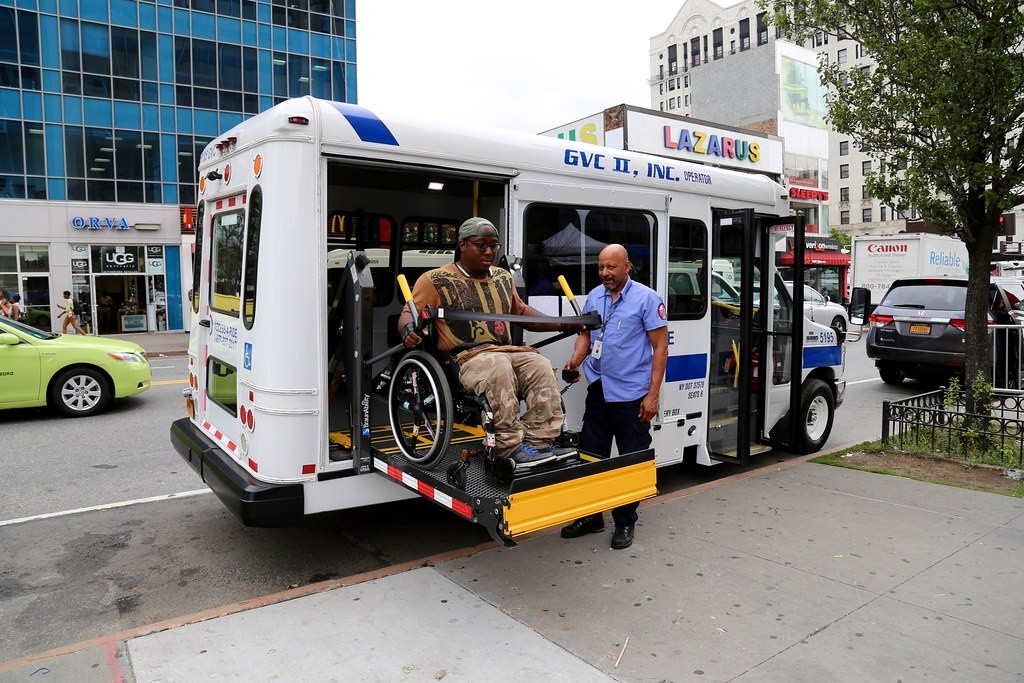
[{"x1": 168, "y1": 93, "x2": 872, "y2": 535}]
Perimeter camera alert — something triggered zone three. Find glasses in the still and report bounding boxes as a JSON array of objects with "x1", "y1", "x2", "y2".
[{"x1": 466, "y1": 238, "x2": 502, "y2": 253}]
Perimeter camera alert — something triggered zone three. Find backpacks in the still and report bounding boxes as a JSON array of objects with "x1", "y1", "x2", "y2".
[{"x1": 69, "y1": 298, "x2": 82, "y2": 314}]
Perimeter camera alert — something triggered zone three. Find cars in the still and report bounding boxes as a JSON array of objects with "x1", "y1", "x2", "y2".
[
  {"x1": 2, "y1": 317, "x2": 153, "y2": 416},
  {"x1": 717, "y1": 280, "x2": 850, "y2": 344}
]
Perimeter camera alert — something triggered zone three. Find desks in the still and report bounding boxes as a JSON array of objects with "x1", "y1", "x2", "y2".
[{"x1": 117, "y1": 309, "x2": 146, "y2": 333}]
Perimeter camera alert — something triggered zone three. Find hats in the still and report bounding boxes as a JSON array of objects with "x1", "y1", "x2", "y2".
[{"x1": 455, "y1": 216, "x2": 500, "y2": 262}]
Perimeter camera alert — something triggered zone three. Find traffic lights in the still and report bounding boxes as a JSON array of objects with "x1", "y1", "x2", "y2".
[{"x1": 998, "y1": 215, "x2": 1006, "y2": 235}]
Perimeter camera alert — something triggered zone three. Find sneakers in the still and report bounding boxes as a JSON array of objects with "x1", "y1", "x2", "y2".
[
  {"x1": 538, "y1": 444, "x2": 580, "y2": 463},
  {"x1": 510, "y1": 443, "x2": 557, "y2": 467}
]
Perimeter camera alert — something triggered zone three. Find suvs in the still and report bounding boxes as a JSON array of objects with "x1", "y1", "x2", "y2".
[{"x1": 865, "y1": 277, "x2": 1024, "y2": 385}]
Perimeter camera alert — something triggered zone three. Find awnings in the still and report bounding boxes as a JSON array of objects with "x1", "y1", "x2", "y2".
[{"x1": 781, "y1": 251, "x2": 852, "y2": 266}]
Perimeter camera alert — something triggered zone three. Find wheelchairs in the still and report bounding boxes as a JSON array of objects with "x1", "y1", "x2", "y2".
[{"x1": 386, "y1": 304, "x2": 579, "y2": 490}]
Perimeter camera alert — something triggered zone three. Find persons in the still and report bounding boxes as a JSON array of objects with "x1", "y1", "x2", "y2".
[
  {"x1": 0, "y1": 289, "x2": 23, "y2": 321},
  {"x1": 56, "y1": 291, "x2": 86, "y2": 336},
  {"x1": 98, "y1": 291, "x2": 112, "y2": 327},
  {"x1": 559, "y1": 244, "x2": 669, "y2": 549},
  {"x1": 398, "y1": 217, "x2": 587, "y2": 470}
]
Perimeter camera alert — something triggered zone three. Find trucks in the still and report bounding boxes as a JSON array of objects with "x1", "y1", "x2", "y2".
[{"x1": 848, "y1": 232, "x2": 971, "y2": 321}]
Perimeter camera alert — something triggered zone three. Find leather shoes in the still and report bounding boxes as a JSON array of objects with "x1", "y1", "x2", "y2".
[
  {"x1": 561, "y1": 516, "x2": 605, "y2": 538},
  {"x1": 611, "y1": 524, "x2": 636, "y2": 549}
]
